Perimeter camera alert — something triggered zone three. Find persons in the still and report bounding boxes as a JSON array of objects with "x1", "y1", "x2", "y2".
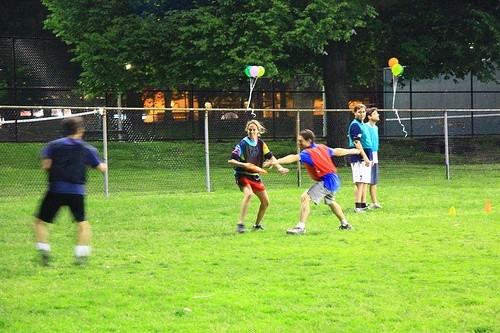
[
  {"x1": 32, "y1": 117, "x2": 107, "y2": 266},
  {"x1": 349, "y1": 104, "x2": 375, "y2": 213},
  {"x1": 365, "y1": 107, "x2": 381, "y2": 208},
  {"x1": 263, "y1": 129, "x2": 361, "y2": 234},
  {"x1": 227, "y1": 119, "x2": 290, "y2": 233}
]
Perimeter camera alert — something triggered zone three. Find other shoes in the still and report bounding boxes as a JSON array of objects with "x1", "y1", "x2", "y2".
[
  {"x1": 253, "y1": 226, "x2": 264, "y2": 231},
  {"x1": 286, "y1": 227, "x2": 305, "y2": 235},
  {"x1": 336, "y1": 223, "x2": 353, "y2": 230},
  {"x1": 354, "y1": 208, "x2": 364, "y2": 212},
  {"x1": 364, "y1": 206, "x2": 371, "y2": 211},
  {"x1": 39, "y1": 251, "x2": 48, "y2": 266},
  {"x1": 75, "y1": 256, "x2": 89, "y2": 267},
  {"x1": 373, "y1": 203, "x2": 382, "y2": 209},
  {"x1": 237, "y1": 226, "x2": 246, "y2": 233}
]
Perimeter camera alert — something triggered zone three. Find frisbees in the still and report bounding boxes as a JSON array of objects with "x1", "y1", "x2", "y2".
[{"x1": 248, "y1": 165, "x2": 267, "y2": 174}]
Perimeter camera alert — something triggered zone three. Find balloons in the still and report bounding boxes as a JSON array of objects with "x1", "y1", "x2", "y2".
[
  {"x1": 245, "y1": 65, "x2": 265, "y2": 78},
  {"x1": 388, "y1": 58, "x2": 404, "y2": 78}
]
